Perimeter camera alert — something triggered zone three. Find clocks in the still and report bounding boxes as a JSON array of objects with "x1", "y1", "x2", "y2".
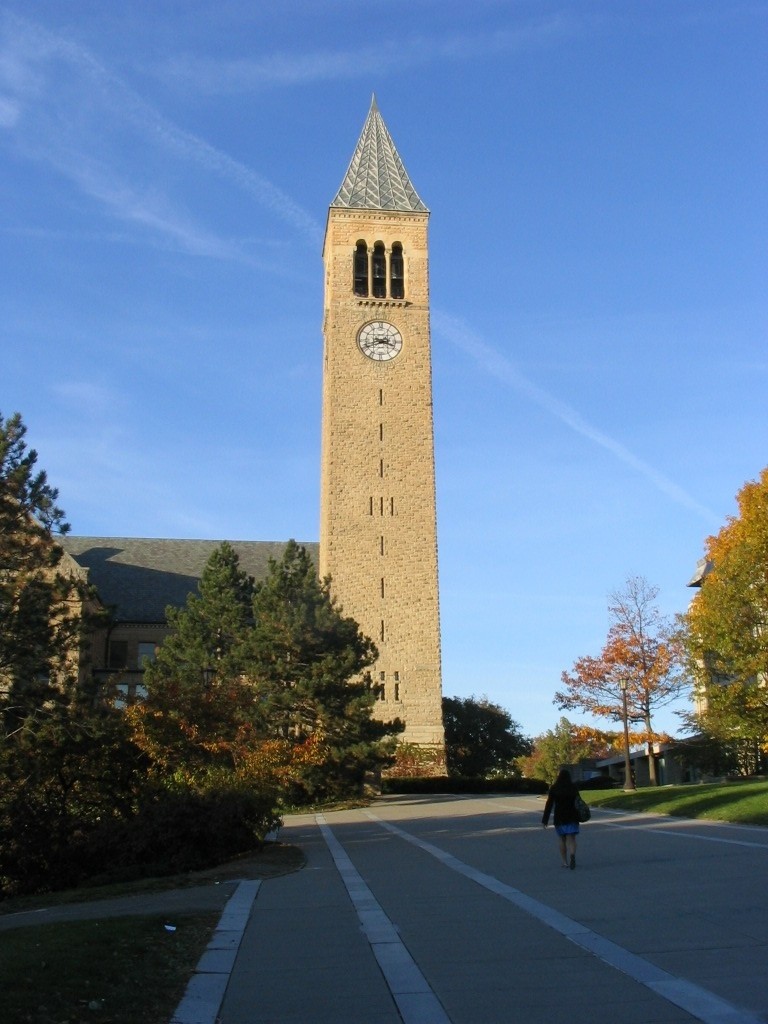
[{"x1": 359, "y1": 321, "x2": 402, "y2": 360}]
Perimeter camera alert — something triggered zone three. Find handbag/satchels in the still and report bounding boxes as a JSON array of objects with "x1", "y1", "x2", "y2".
[{"x1": 570, "y1": 784, "x2": 591, "y2": 822}]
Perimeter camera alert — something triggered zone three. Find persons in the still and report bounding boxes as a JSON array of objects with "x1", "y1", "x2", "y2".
[{"x1": 541, "y1": 769, "x2": 581, "y2": 870}]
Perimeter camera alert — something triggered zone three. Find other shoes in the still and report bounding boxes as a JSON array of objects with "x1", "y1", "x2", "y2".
[
  {"x1": 560, "y1": 863, "x2": 568, "y2": 868},
  {"x1": 570, "y1": 854, "x2": 575, "y2": 870}
]
